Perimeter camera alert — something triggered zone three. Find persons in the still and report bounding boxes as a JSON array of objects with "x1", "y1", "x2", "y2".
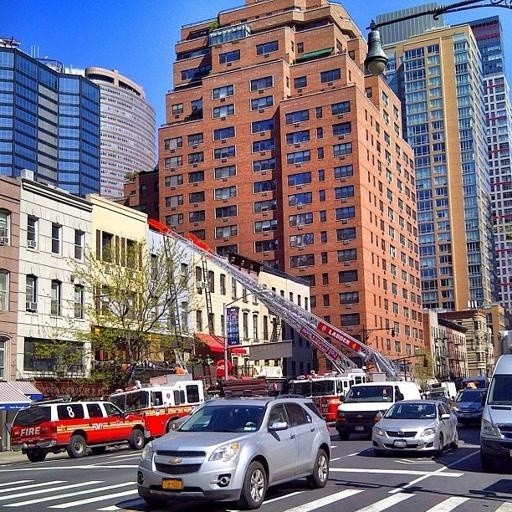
[
  {"x1": 420, "y1": 405, "x2": 436, "y2": 418},
  {"x1": 383, "y1": 389, "x2": 392, "y2": 396}
]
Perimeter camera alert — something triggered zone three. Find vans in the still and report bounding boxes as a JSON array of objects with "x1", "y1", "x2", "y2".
[{"x1": 336, "y1": 329, "x2": 512, "y2": 467}]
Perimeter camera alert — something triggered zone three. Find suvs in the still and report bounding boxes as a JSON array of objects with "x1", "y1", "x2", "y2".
[{"x1": 9, "y1": 399, "x2": 150, "y2": 461}]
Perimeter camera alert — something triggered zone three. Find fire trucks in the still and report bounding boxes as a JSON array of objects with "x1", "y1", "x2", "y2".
[
  {"x1": 146, "y1": 216, "x2": 416, "y2": 428},
  {"x1": 106, "y1": 371, "x2": 271, "y2": 440}
]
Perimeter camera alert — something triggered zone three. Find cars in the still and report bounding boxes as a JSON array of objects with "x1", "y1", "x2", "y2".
[{"x1": 135, "y1": 399, "x2": 333, "y2": 510}]
